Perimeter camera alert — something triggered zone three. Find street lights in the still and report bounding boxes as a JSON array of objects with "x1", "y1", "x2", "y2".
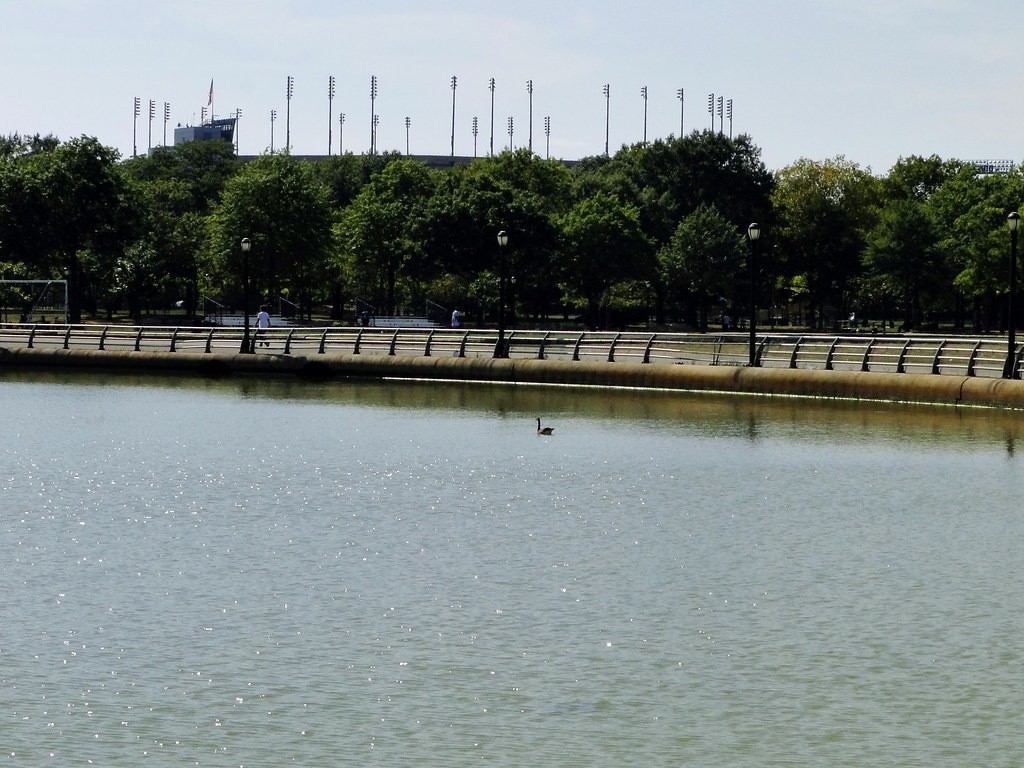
[
  {"x1": 1002, "y1": 211, "x2": 1021, "y2": 378},
  {"x1": 239, "y1": 234, "x2": 253, "y2": 352},
  {"x1": 492, "y1": 228, "x2": 512, "y2": 358},
  {"x1": 746, "y1": 222, "x2": 764, "y2": 365}
]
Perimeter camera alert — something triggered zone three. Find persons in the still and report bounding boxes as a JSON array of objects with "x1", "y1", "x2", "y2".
[
  {"x1": 361, "y1": 307, "x2": 369, "y2": 333},
  {"x1": 451, "y1": 306, "x2": 463, "y2": 333},
  {"x1": 252, "y1": 305, "x2": 273, "y2": 347},
  {"x1": 19, "y1": 312, "x2": 65, "y2": 330},
  {"x1": 848, "y1": 312, "x2": 855, "y2": 328},
  {"x1": 721, "y1": 312, "x2": 732, "y2": 331}
]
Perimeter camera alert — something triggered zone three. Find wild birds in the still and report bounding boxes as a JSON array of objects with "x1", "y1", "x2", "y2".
[{"x1": 535, "y1": 417, "x2": 555, "y2": 435}]
